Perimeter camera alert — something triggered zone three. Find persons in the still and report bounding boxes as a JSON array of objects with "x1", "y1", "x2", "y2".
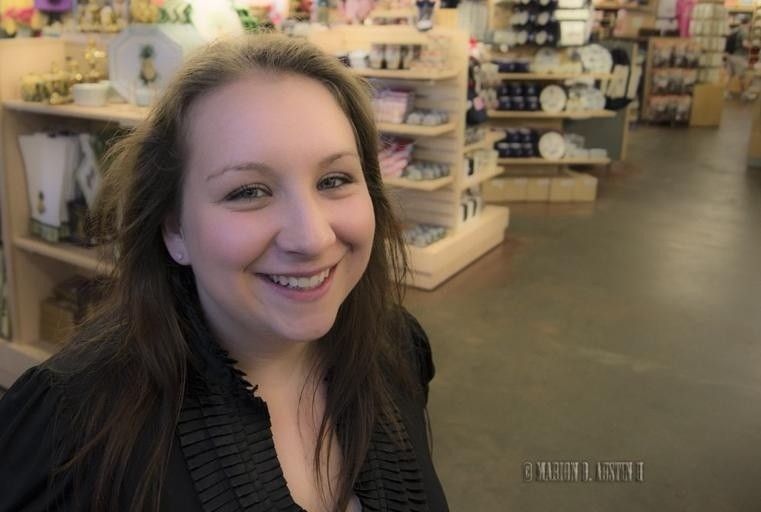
[{"x1": 0, "y1": 34, "x2": 449, "y2": 512}]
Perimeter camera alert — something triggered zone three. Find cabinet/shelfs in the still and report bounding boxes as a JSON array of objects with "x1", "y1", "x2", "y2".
[
  {"x1": 465, "y1": 0, "x2": 761, "y2": 264},
  {"x1": 1, "y1": 34, "x2": 184, "y2": 364},
  {"x1": 299, "y1": 16, "x2": 466, "y2": 291}
]
